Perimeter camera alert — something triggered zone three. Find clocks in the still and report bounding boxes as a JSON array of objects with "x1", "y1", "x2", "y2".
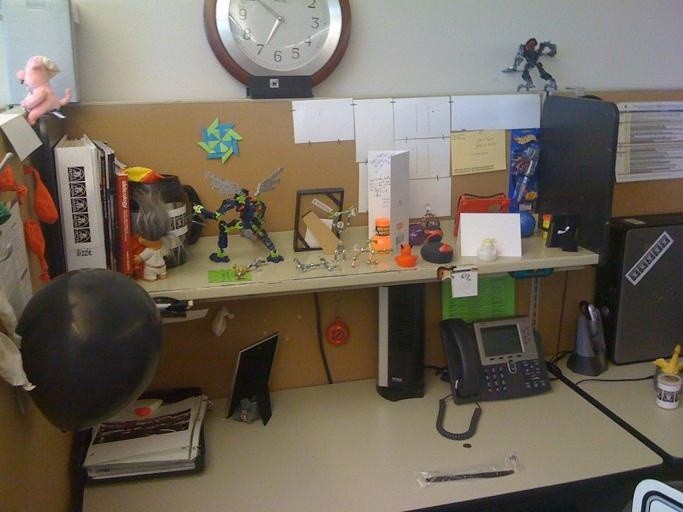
[{"x1": 203, "y1": 0, "x2": 351, "y2": 98}]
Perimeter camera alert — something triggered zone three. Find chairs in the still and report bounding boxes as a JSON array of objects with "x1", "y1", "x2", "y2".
[{"x1": 632, "y1": 479, "x2": 683, "y2": 512}]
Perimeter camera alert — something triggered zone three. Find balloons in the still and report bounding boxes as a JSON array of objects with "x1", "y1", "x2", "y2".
[
  {"x1": 17, "y1": 269, "x2": 163, "y2": 434},
  {"x1": 0, "y1": 161, "x2": 27, "y2": 204},
  {"x1": 22, "y1": 163, "x2": 60, "y2": 225},
  {"x1": 23, "y1": 218, "x2": 51, "y2": 281}
]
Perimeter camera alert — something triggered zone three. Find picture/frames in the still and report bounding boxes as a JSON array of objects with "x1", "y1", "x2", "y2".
[
  {"x1": 293, "y1": 187, "x2": 344, "y2": 252},
  {"x1": 226, "y1": 333, "x2": 280, "y2": 426}
]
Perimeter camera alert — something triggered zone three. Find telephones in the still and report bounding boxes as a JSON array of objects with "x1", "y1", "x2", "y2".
[{"x1": 440, "y1": 316, "x2": 550, "y2": 404}]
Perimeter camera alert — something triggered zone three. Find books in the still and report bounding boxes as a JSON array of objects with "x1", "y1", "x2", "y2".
[
  {"x1": 71, "y1": 384, "x2": 212, "y2": 486},
  {"x1": 54, "y1": 132, "x2": 135, "y2": 280}
]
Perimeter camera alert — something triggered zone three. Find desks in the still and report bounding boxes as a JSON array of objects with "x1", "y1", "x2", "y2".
[
  {"x1": 546, "y1": 353, "x2": 683, "y2": 466},
  {"x1": 80, "y1": 354, "x2": 663, "y2": 512}
]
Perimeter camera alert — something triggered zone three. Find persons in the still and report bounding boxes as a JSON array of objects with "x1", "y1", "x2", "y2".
[
  {"x1": 502, "y1": 37, "x2": 560, "y2": 94},
  {"x1": 130, "y1": 187, "x2": 173, "y2": 281},
  {"x1": 191, "y1": 165, "x2": 292, "y2": 265}
]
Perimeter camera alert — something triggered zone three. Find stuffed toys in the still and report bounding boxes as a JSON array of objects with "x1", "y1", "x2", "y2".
[{"x1": 14, "y1": 54, "x2": 72, "y2": 125}]
